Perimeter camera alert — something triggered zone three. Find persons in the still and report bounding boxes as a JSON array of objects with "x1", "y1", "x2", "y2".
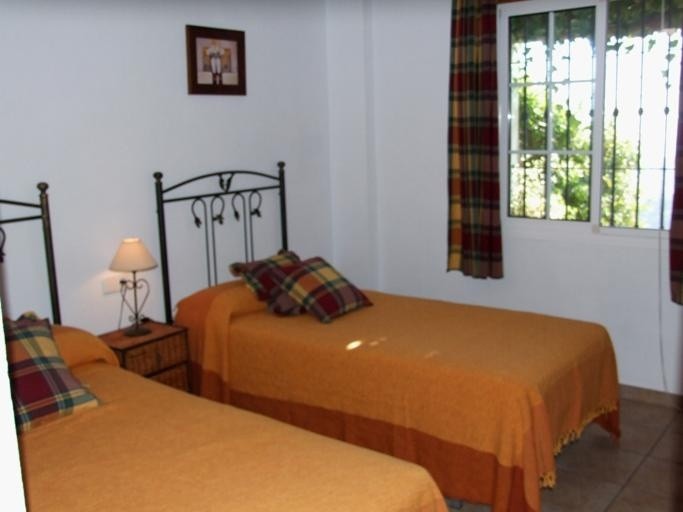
[{"x1": 204, "y1": 39, "x2": 226, "y2": 86}]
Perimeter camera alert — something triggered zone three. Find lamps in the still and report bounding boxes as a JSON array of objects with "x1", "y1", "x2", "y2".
[{"x1": 110, "y1": 237, "x2": 158, "y2": 336}]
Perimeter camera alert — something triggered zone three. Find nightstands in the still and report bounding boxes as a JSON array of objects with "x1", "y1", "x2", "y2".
[{"x1": 98, "y1": 322, "x2": 194, "y2": 394}]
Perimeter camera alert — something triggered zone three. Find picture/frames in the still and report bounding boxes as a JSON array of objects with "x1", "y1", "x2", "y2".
[{"x1": 186, "y1": 26, "x2": 245, "y2": 95}]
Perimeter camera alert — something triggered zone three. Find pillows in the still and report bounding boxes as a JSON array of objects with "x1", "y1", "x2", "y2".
[
  {"x1": 4, "y1": 311, "x2": 120, "y2": 434},
  {"x1": 177, "y1": 250, "x2": 373, "y2": 324}
]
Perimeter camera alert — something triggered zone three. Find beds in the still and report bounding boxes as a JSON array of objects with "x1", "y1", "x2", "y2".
[
  {"x1": 153, "y1": 162, "x2": 619, "y2": 512},
  {"x1": 0, "y1": 183, "x2": 447, "y2": 512}
]
